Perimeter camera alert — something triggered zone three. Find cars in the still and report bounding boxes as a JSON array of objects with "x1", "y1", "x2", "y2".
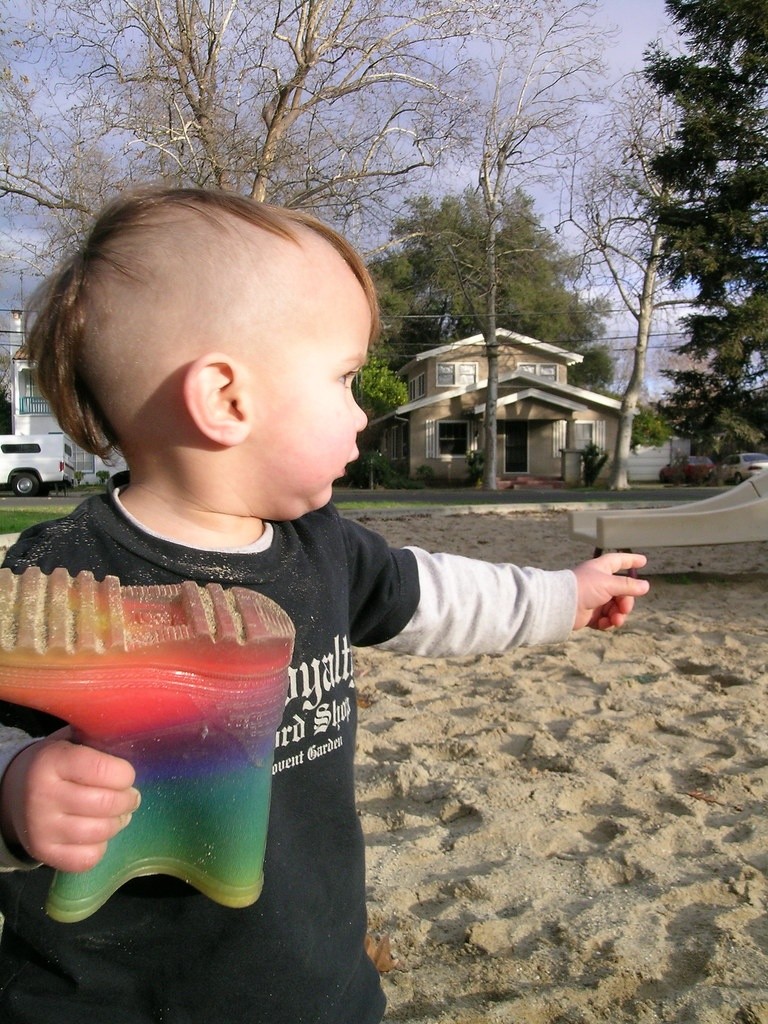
[
  {"x1": 659, "y1": 455, "x2": 717, "y2": 484},
  {"x1": 708, "y1": 452, "x2": 768, "y2": 485}
]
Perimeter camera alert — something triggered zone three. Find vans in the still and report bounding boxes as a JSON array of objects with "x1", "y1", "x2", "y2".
[{"x1": 0, "y1": 433, "x2": 76, "y2": 497}]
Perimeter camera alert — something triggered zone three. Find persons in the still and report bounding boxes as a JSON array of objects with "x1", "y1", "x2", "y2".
[{"x1": 0, "y1": 189, "x2": 649, "y2": 1024}]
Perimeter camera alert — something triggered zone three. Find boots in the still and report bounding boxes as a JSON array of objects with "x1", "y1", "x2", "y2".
[{"x1": 0, "y1": 567, "x2": 296, "y2": 923}]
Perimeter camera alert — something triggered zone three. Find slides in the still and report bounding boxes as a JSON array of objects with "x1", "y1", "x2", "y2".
[{"x1": 562, "y1": 465, "x2": 768, "y2": 552}]
